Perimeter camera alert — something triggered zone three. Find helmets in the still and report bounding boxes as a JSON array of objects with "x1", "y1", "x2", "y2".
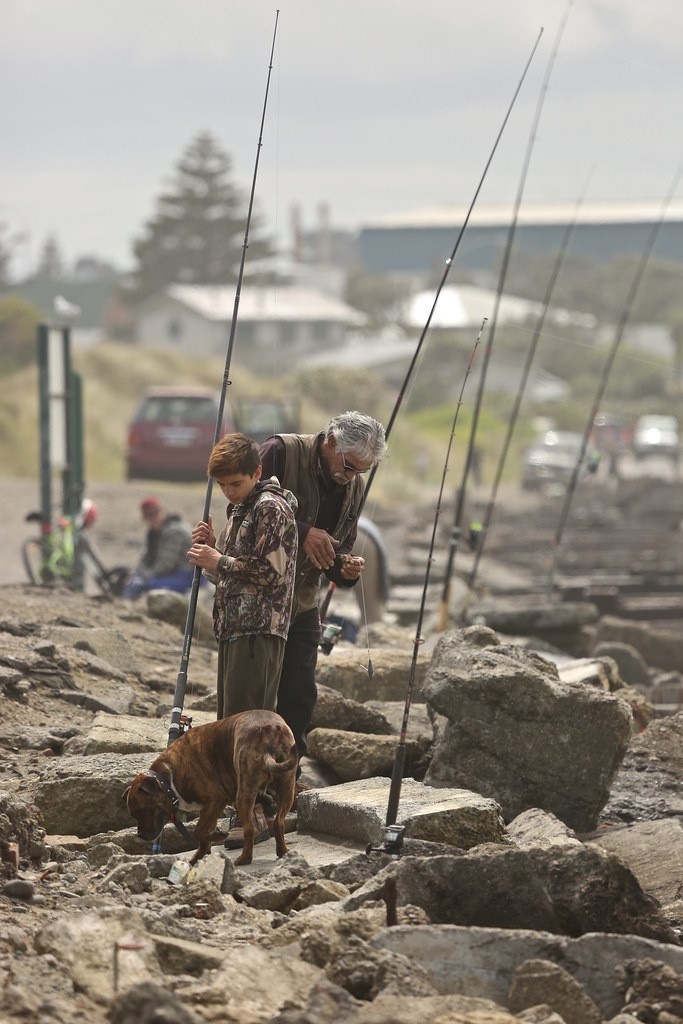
[{"x1": 74, "y1": 499, "x2": 97, "y2": 529}]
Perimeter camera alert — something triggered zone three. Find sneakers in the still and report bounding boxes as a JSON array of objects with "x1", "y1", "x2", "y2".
[
  {"x1": 225, "y1": 803, "x2": 269, "y2": 848},
  {"x1": 266, "y1": 815, "x2": 277, "y2": 836}
]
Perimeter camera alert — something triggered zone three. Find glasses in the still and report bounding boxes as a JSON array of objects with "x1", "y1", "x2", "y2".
[{"x1": 341, "y1": 448, "x2": 371, "y2": 473}]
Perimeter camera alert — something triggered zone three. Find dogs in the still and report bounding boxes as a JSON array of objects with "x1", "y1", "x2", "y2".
[{"x1": 121, "y1": 709, "x2": 302, "y2": 865}]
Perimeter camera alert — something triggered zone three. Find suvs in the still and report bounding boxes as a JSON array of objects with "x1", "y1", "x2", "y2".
[{"x1": 127, "y1": 386, "x2": 235, "y2": 483}]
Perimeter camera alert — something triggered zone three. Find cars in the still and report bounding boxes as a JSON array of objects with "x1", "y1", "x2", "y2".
[
  {"x1": 520, "y1": 432, "x2": 594, "y2": 493},
  {"x1": 633, "y1": 413, "x2": 680, "y2": 459}
]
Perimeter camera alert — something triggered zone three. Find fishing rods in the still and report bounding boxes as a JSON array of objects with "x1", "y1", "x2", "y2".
[
  {"x1": 458, "y1": 222, "x2": 576, "y2": 627},
  {"x1": 149, "y1": 4, "x2": 282, "y2": 854},
  {"x1": 361, "y1": 314, "x2": 488, "y2": 926},
  {"x1": 553, "y1": 165, "x2": 682, "y2": 545},
  {"x1": 435, "y1": 0, "x2": 575, "y2": 633},
  {"x1": 318, "y1": 24, "x2": 546, "y2": 620}
]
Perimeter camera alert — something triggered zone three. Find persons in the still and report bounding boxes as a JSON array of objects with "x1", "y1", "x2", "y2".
[
  {"x1": 187, "y1": 432, "x2": 299, "y2": 849},
  {"x1": 225, "y1": 411, "x2": 388, "y2": 837},
  {"x1": 122, "y1": 499, "x2": 194, "y2": 600}
]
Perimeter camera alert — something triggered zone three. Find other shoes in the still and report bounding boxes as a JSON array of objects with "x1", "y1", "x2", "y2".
[{"x1": 292, "y1": 780, "x2": 312, "y2": 812}]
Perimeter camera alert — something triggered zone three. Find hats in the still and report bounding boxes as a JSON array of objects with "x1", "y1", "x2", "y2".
[{"x1": 140, "y1": 497, "x2": 161, "y2": 514}]
